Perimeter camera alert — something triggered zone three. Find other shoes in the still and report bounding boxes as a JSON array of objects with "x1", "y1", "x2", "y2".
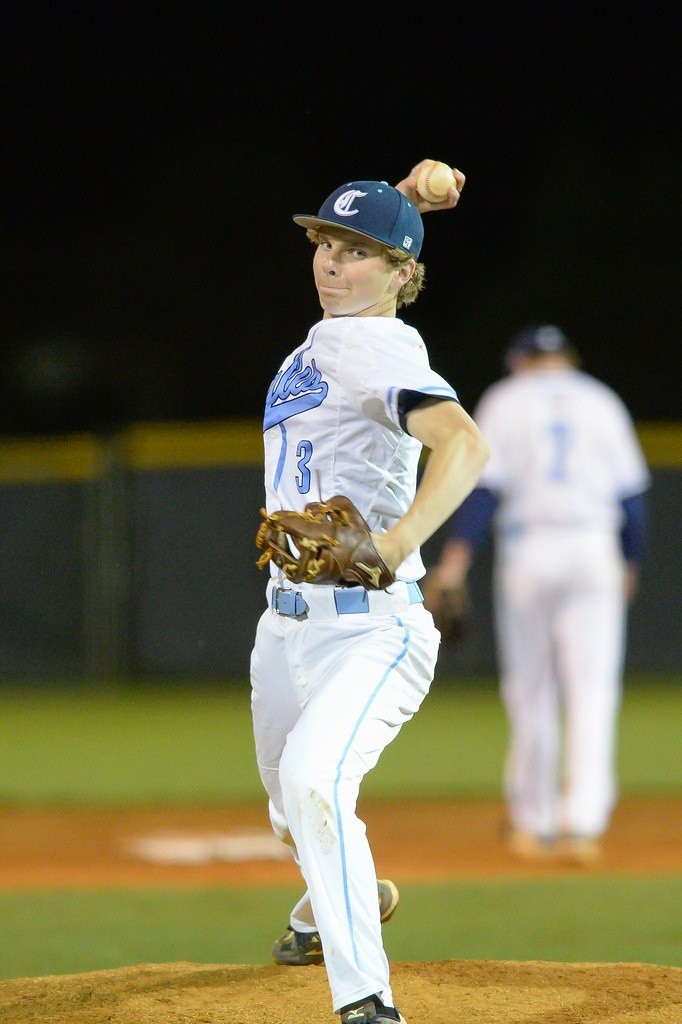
[
  {"x1": 510, "y1": 835, "x2": 553, "y2": 870},
  {"x1": 556, "y1": 833, "x2": 600, "y2": 869}
]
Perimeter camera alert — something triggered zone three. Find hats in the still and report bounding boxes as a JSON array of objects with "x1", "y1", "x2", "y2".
[
  {"x1": 293, "y1": 181, "x2": 424, "y2": 262},
  {"x1": 512, "y1": 323, "x2": 569, "y2": 355}
]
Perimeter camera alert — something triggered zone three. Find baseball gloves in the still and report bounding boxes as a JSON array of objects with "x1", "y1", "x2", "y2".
[{"x1": 253, "y1": 495, "x2": 396, "y2": 594}]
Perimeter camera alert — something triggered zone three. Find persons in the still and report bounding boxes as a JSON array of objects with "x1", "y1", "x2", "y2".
[
  {"x1": 251, "y1": 158, "x2": 490, "y2": 1024},
  {"x1": 427, "y1": 318, "x2": 651, "y2": 863}
]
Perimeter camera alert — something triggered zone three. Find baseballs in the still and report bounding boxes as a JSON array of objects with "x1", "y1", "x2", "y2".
[{"x1": 416, "y1": 161, "x2": 457, "y2": 203}]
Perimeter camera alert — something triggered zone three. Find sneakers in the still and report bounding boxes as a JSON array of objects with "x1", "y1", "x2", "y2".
[
  {"x1": 269, "y1": 878, "x2": 399, "y2": 966},
  {"x1": 339, "y1": 996, "x2": 408, "y2": 1024}
]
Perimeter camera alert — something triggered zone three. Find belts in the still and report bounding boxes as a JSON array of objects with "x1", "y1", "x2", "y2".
[{"x1": 271, "y1": 581, "x2": 425, "y2": 619}]
